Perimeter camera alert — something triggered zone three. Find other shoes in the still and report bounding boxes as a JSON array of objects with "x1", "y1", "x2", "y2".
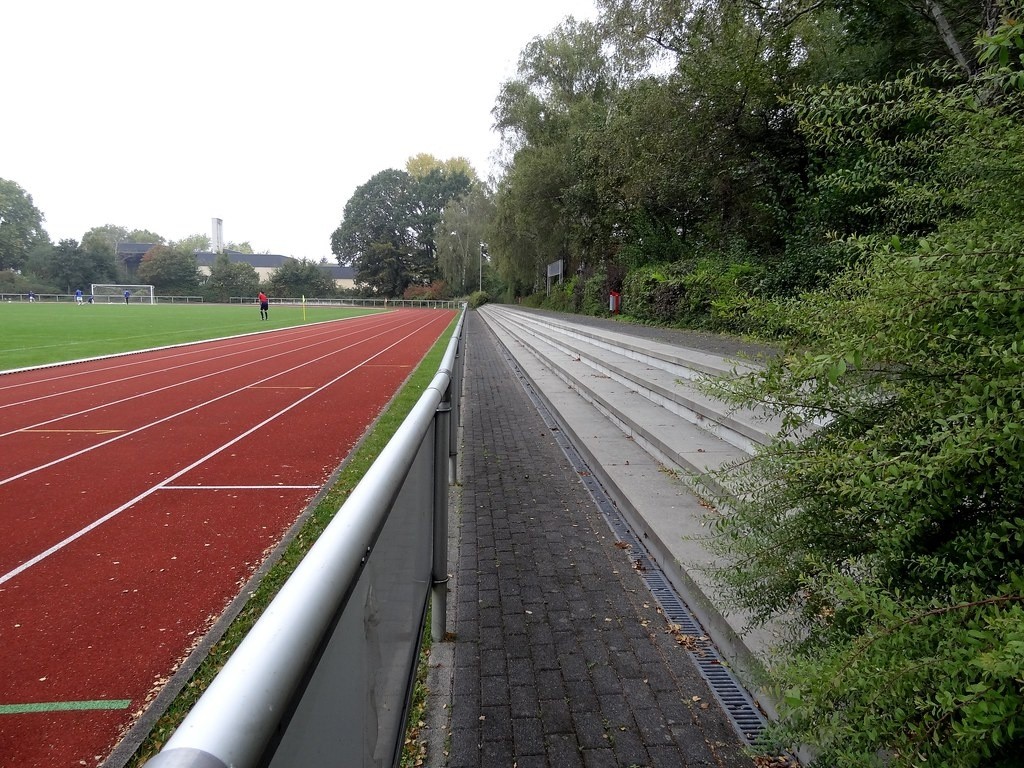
[
  {"x1": 260, "y1": 319, "x2": 264, "y2": 320},
  {"x1": 266, "y1": 319, "x2": 268, "y2": 320}
]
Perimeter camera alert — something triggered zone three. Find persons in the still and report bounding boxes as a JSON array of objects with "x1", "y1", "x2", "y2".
[
  {"x1": 88, "y1": 296, "x2": 93, "y2": 303},
  {"x1": 29, "y1": 291, "x2": 34, "y2": 303},
  {"x1": 75, "y1": 289, "x2": 84, "y2": 306},
  {"x1": 124, "y1": 289, "x2": 129, "y2": 304},
  {"x1": 258, "y1": 292, "x2": 268, "y2": 320}
]
